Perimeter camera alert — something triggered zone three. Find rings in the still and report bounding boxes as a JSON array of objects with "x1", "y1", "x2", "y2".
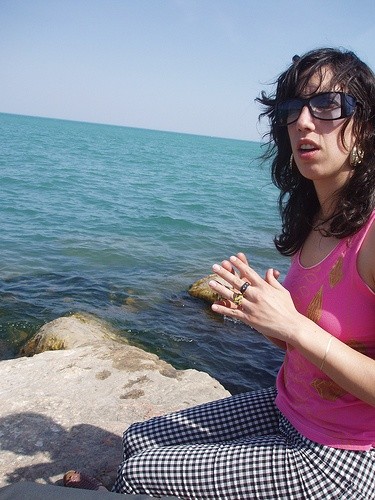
[{"x1": 239, "y1": 279, "x2": 251, "y2": 294}]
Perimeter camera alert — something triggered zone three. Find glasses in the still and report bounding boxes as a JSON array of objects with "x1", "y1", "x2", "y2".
[{"x1": 274, "y1": 92, "x2": 363, "y2": 126}]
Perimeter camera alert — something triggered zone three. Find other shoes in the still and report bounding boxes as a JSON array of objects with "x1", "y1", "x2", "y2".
[{"x1": 63, "y1": 470, "x2": 119, "y2": 490}]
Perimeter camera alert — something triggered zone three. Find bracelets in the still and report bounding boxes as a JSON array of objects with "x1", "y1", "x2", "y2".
[{"x1": 316, "y1": 331, "x2": 336, "y2": 372}]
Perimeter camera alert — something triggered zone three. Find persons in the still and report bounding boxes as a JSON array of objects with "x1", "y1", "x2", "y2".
[{"x1": 117, "y1": 47, "x2": 375, "y2": 500}]
